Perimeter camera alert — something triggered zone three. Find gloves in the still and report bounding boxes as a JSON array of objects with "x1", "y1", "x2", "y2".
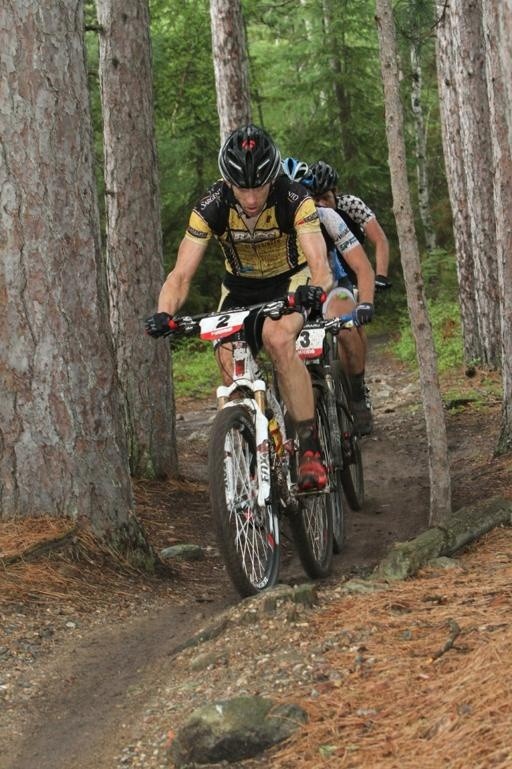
[
  {"x1": 144, "y1": 311, "x2": 174, "y2": 340},
  {"x1": 374, "y1": 275, "x2": 388, "y2": 294},
  {"x1": 293, "y1": 284, "x2": 324, "y2": 311},
  {"x1": 352, "y1": 302, "x2": 375, "y2": 327}
]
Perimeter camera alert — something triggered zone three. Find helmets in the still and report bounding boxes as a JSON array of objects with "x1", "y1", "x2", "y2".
[
  {"x1": 217, "y1": 124, "x2": 281, "y2": 189},
  {"x1": 282, "y1": 156, "x2": 340, "y2": 198}
]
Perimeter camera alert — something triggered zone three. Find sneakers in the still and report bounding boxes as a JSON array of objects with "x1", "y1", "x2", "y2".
[
  {"x1": 350, "y1": 399, "x2": 374, "y2": 435},
  {"x1": 297, "y1": 448, "x2": 328, "y2": 491}
]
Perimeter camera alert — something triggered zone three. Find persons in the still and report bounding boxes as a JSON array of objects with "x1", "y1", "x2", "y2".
[
  {"x1": 279, "y1": 156, "x2": 378, "y2": 436},
  {"x1": 311, "y1": 160, "x2": 389, "y2": 394},
  {"x1": 144, "y1": 122, "x2": 333, "y2": 530}
]
